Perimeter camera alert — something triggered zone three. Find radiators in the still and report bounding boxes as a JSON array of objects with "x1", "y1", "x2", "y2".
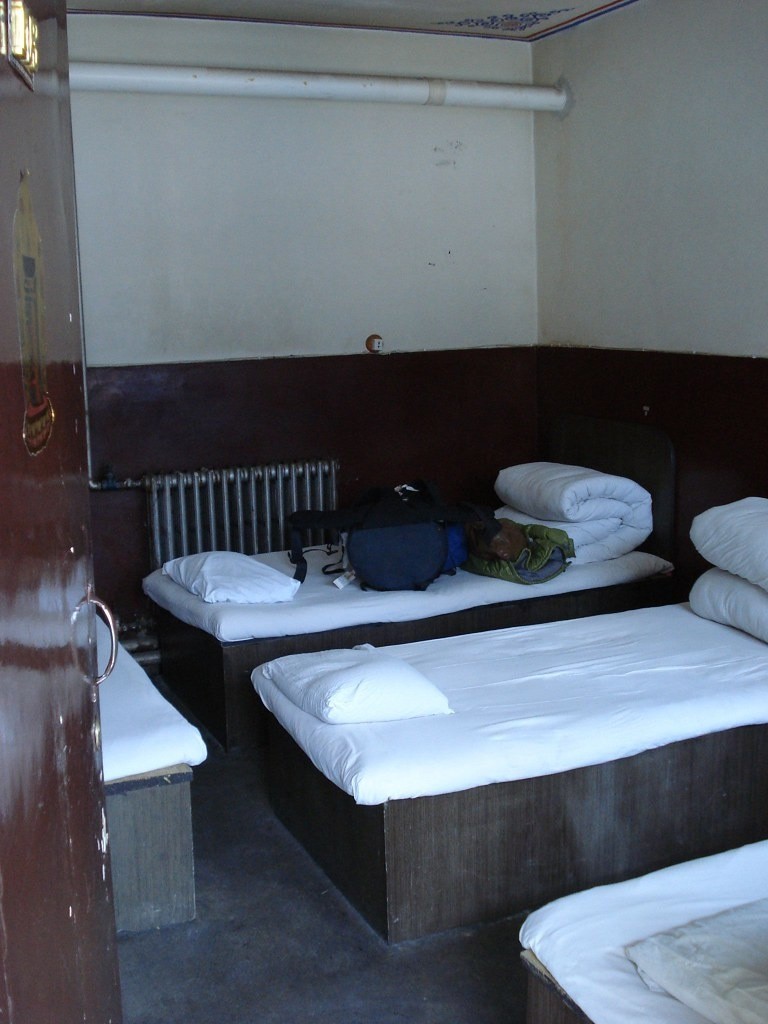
[{"x1": 148, "y1": 459, "x2": 338, "y2": 571}]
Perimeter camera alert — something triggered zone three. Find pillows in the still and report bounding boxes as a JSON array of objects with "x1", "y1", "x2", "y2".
[
  {"x1": 261, "y1": 643, "x2": 455, "y2": 725},
  {"x1": 162, "y1": 551, "x2": 301, "y2": 603}
]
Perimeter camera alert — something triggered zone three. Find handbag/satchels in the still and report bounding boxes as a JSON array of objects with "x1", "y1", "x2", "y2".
[{"x1": 447, "y1": 500, "x2": 529, "y2": 562}]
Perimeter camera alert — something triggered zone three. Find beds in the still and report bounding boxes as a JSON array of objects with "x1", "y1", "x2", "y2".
[
  {"x1": 97, "y1": 612, "x2": 209, "y2": 937},
  {"x1": 250, "y1": 603, "x2": 768, "y2": 947},
  {"x1": 518, "y1": 839, "x2": 768, "y2": 1024},
  {"x1": 141, "y1": 542, "x2": 675, "y2": 760}
]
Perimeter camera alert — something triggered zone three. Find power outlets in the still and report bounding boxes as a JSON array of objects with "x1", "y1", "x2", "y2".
[{"x1": 366, "y1": 335, "x2": 385, "y2": 352}]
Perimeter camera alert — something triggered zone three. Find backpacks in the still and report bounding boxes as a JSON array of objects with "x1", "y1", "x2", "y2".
[{"x1": 286, "y1": 479, "x2": 467, "y2": 591}]
